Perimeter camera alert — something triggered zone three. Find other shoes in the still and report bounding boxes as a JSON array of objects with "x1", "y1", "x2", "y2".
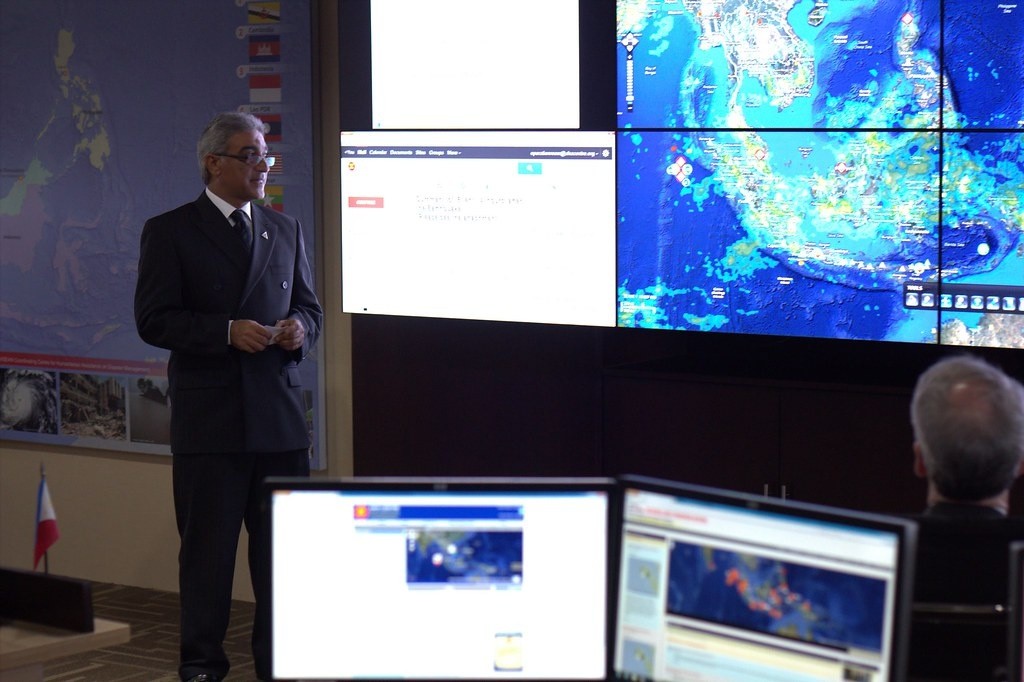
[{"x1": 188, "y1": 674, "x2": 216, "y2": 682}]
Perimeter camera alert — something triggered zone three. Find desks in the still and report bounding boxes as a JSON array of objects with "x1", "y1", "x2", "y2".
[{"x1": 0, "y1": 607, "x2": 131, "y2": 668}]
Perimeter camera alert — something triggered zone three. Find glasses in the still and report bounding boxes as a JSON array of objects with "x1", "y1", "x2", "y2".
[{"x1": 210, "y1": 153, "x2": 275, "y2": 167}]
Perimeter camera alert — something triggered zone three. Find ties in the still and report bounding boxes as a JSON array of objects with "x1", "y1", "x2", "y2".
[{"x1": 229, "y1": 210, "x2": 254, "y2": 255}]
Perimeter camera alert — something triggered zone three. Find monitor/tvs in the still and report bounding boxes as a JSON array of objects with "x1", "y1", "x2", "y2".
[
  {"x1": 610, "y1": 474, "x2": 920, "y2": 682},
  {"x1": 260, "y1": 476, "x2": 620, "y2": 682}
]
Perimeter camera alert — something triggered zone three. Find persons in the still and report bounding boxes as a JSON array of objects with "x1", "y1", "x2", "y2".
[
  {"x1": 880, "y1": 353, "x2": 1024, "y2": 682},
  {"x1": 132, "y1": 111, "x2": 325, "y2": 681}
]
